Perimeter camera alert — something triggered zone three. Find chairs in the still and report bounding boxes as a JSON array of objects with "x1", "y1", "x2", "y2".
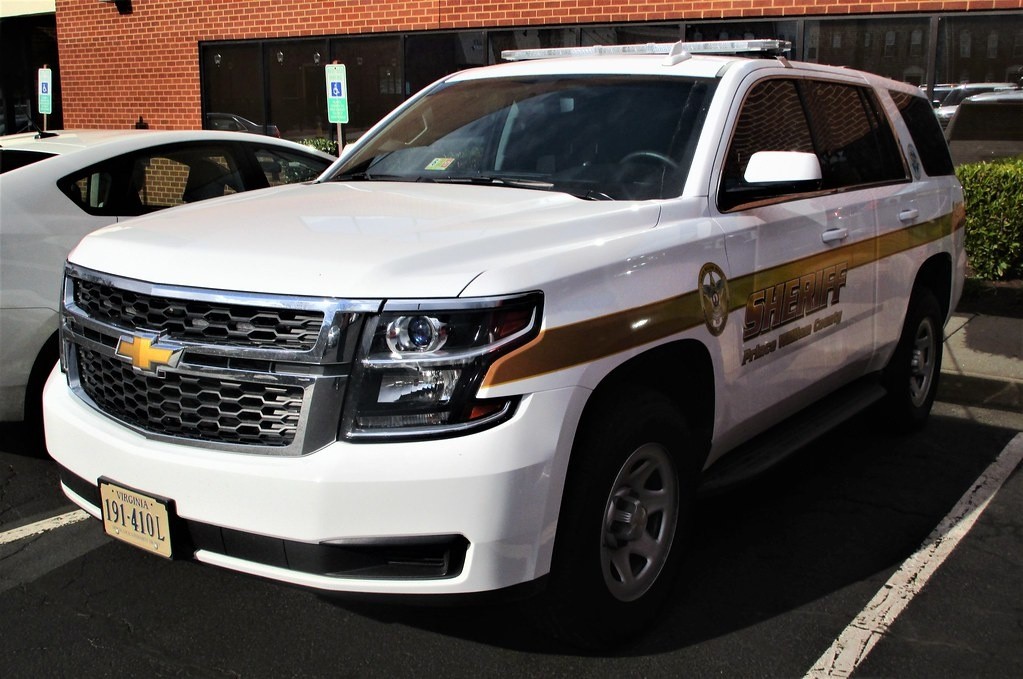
[
  {"x1": 101, "y1": 160, "x2": 145, "y2": 213},
  {"x1": 181, "y1": 160, "x2": 225, "y2": 203}
]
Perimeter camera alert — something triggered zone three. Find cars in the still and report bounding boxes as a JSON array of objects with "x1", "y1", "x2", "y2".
[
  {"x1": 917, "y1": 79, "x2": 1023, "y2": 166},
  {"x1": 1, "y1": 122, "x2": 342, "y2": 461},
  {"x1": 206, "y1": 111, "x2": 281, "y2": 139}
]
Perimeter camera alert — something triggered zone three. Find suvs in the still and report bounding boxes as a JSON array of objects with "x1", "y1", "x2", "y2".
[{"x1": 37, "y1": 36, "x2": 967, "y2": 624}]
